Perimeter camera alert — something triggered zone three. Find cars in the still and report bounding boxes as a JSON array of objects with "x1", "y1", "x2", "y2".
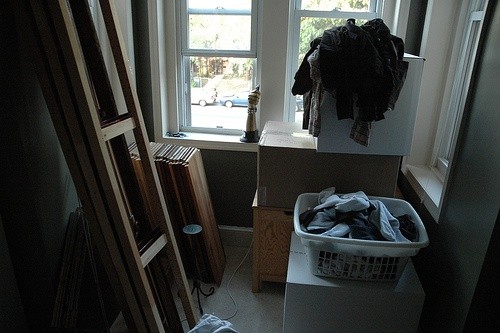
[
  {"x1": 220, "y1": 94, "x2": 252, "y2": 108},
  {"x1": 191, "y1": 87, "x2": 218, "y2": 106}
]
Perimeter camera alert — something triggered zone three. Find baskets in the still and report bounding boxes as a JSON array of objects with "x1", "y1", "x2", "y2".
[{"x1": 294, "y1": 193, "x2": 429, "y2": 281}]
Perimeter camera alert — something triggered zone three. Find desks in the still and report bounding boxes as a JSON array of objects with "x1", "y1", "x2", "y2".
[{"x1": 251, "y1": 187, "x2": 403, "y2": 296}]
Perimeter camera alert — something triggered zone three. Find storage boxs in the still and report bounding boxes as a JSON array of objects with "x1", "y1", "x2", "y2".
[
  {"x1": 258, "y1": 120, "x2": 401, "y2": 209},
  {"x1": 294, "y1": 192, "x2": 430, "y2": 283},
  {"x1": 315, "y1": 52, "x2": 426, "y2": 157},
  {"x1": 281, "y1": 231, "x2": 426, "y2": 333}
]
